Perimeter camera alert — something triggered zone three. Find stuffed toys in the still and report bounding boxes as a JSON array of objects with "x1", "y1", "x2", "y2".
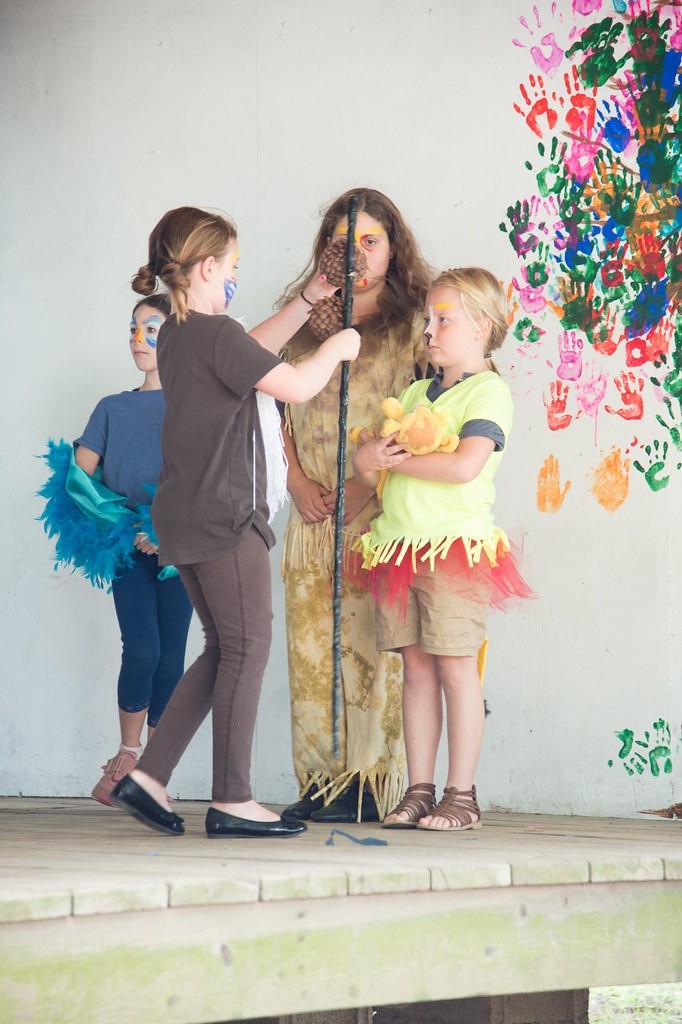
[{"x1": 349, "y1": 396, "x2": 460, "y2": 457}]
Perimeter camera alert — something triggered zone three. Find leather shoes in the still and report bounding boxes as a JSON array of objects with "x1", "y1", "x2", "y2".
[
  {"x1": 311, "y1": 779, "x2": 379, "y2": 821},
  {"x1": 110, "y1": 775, "x2": 185, "y2": 834},
  {"x1": 281, "y1": 781, "x2": 332, "y2": 819},
  {"x1": 205, "y1": 805, "x2": 307, "y2": 839}
]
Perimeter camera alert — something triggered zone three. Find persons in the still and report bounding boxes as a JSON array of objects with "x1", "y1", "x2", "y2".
[
  {"x1": 112, "y1": 207, "x2": 361, "y2": 839},
  {"x1": 273, "y1": 188, "x2": 436, "y2": 822},
  {"x1": 32, "y1": 294, "x2": 192, "y2": 806},
  {"x1": 354, "y1": 267, "x2": 512, "y2": 832}
]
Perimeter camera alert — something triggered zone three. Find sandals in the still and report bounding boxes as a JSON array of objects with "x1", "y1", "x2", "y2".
[
  {"x1": 416, "y1": 785, "x2": 482, "y2": 830},
  {"x1": 381, "y1": 783, "x2": 436, "y2": 828}
]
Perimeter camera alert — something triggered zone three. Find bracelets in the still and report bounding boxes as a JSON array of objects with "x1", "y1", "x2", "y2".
[{"x1": 300, "y1": 289, "x2": 318, "y2": 306}]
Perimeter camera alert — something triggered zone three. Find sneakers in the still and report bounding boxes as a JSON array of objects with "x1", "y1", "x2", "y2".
[{"x1": 91, "y1": 749, "x2": 140, "y2": 806}]
